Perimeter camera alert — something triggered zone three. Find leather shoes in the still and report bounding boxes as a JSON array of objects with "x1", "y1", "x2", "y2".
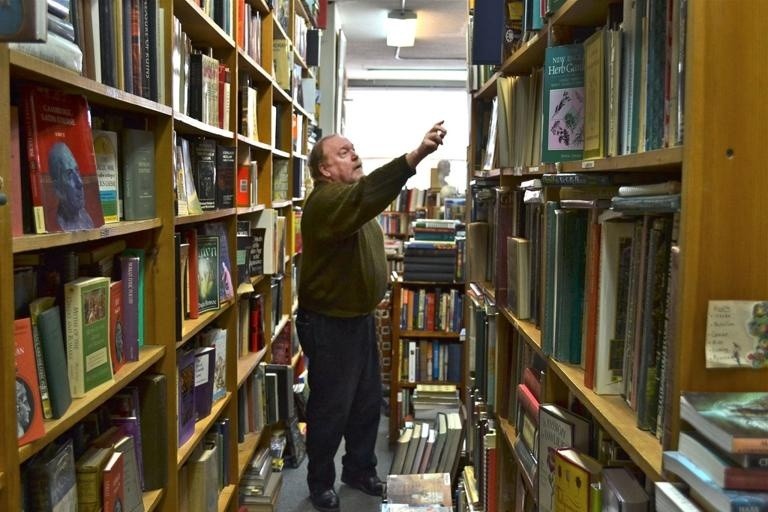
[
  {"x1": 309, "y1": 485, "x2": 341, "y2": 512},
  {"x1": 340, "y1": 466, "x2": 388, "y2": 496}
]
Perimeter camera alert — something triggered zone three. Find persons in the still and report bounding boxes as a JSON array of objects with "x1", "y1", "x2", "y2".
[
  {"x1": 292, "y1": 121, "x2": 447, "y2": 510},
  {"x1": 437, "y1": 160, "x2": 450, "y2": 187}
]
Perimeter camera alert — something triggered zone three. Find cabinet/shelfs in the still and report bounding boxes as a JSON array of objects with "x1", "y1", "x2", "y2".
[
  {"x1": 375, "y1": 211, "x2": 466, "y2": 279},
  {"x1": 294, "y1": 0, "x2": 321, "y2": 374},
  {"x1": 175, "y1": 0, "x2": 236, "y2": 512},
  {"x1": 273, "y1": 0, "x2": 294, "y2": 467},
  {"x1": 493, "y1": 0, "x2": 549, "y2": 512},
  {"x1": 1, "y1": 0, "x2": 175, "y2": 512},
  {"x1": 466, "y1": 1, "x2": 492, "y2": 511},
  {"x1": 236, "y1": 0, "x2": 273, "y2": 482},
  {"x1": 549, "y1": 0, "x2": 689, "y2": 512},
  {"x1": 391, "y1": 281, "x2": 466, "y2": 447}
]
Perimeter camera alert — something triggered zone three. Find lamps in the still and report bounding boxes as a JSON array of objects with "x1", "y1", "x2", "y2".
[{"x1": 387, "y1": 0, "x2": 416, "y2": 46}]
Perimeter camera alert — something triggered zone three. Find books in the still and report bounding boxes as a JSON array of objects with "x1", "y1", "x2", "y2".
[
  {"x1": 371, "y1": 189, "x2": 470, "y2": 512},
  {"x1": 4, "y1": 2, "x2": 326, "y2": 511},
  {"x1": 472, "y1": 1, "x2": 768, "y2": 512}
]
